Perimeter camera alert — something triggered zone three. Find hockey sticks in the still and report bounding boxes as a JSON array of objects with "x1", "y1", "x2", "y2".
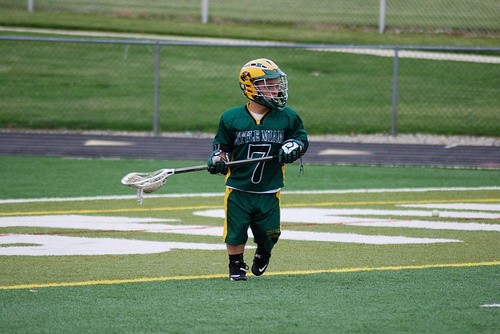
[{"x1": 121, "y1": 145, "x2": 302, "y2": 205}]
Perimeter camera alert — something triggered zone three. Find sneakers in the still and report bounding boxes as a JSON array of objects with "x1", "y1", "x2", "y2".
[
  {"x1": 228, "y1": 259, "x2": 247, "y2": 281},
  {"x1": 251, "y1": 249, "x2": 270, "y2": 276}
]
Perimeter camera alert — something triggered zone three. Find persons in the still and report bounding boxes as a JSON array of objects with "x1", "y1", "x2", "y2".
[{"x1": 208, "y1": 58, "x2": 310, "y2": 282}]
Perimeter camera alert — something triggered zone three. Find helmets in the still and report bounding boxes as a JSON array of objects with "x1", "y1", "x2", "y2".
[{"x1": 239, "y1": 58, "x2": 288, "y2": 111}]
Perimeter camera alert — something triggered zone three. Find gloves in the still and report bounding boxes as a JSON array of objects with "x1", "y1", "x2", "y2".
[
  {"x1": 207, "y1": 150, "x2": 229, "y2": 175},
  {"x1": 277, "y1": 142, "x2": 300, "y2": 164}
]
